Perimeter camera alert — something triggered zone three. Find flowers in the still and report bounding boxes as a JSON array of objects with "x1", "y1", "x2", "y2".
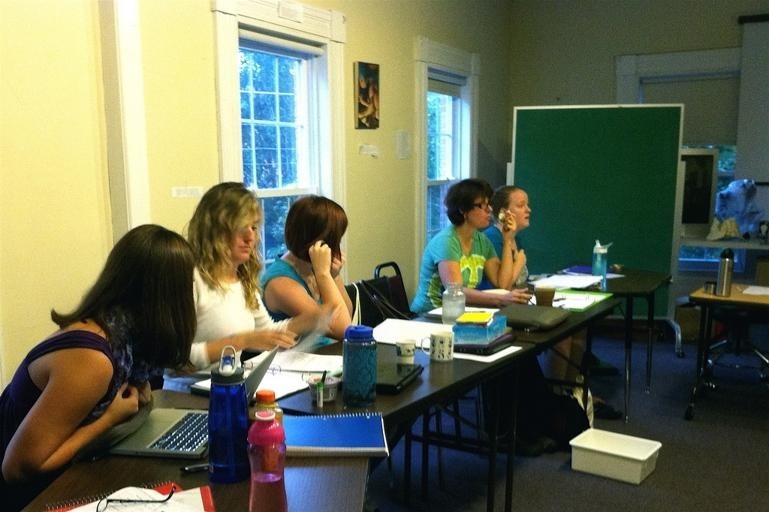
[{"x1": 453, "y1": 253, "x2": 486, "y2": 290}]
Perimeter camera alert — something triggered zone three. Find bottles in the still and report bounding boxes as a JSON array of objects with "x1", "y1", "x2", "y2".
[
  {"x1": 441, "y1": 282, "x2": 466, "y2": 326},
  {"x1": 207, "y1": 345, "x2": 251, "y2": 485},
  {"x1": 342, "y1": 325, "x2": 378, "y2": 408},
  {"x1": 715, "y1": 247, "x2": 734, "y2": 297},
  {"x1": 248, "y1": 390, "x2": 284, "y2": 429},
  {"x1": 591, "y1": 239, "x2": 613, "y2": 291},
  {"x1": 247, "y1": 410, "x2": 288, "y2": 512}
]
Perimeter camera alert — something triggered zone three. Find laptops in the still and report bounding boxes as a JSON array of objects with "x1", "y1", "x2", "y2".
[{"x1": 109, "y1": 341, "x2": 284, "y2": 459}]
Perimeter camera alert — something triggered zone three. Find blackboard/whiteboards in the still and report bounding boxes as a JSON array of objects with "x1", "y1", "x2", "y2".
[{"x1": 509, "y1": 104, "x2": 685, "y2": 320}]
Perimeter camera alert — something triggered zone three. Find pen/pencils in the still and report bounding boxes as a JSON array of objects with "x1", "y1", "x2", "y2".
[{"x1": 182, "y1": 462, "x2": 210, "y2": 472}]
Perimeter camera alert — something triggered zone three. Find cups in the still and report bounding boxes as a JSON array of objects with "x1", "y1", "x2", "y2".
[
  {"x1": 533, "y1": 285, "x2": 556, "y2": 307},
  {"x1": 394, "y1": 363, "x2": 417, "y2": 379},
  {"x1": 421, "y1": 331, "x2": 454, "y2": 362},
  {"x1": 395, "y1": 339, "x2": 416, "y2": 364},
  {"x1": 704, "y1": 281, "x2": 717, "y2": 294},
  {"x1": 306, "y1": 375, "x2": 341, "y2": 403}
]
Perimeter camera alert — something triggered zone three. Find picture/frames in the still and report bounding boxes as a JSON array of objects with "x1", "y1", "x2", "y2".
[{"x1": 353, "y1": 59, "x2": 380, "y2": 130}]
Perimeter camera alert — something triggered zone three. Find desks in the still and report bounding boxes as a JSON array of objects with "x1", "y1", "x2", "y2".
[
  {"x1": 684, "y1": 280, "x2": 769, "y2": 420},
  {"x1": 19, "y1": 264, "x2": 674, "y2": 512}
]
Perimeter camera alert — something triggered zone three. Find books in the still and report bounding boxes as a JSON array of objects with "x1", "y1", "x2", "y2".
[
  {"x1": 44, "y1": 482, "x2": 215, "y2": 512},
  {"x1": 372, "y1": 318, "x2": 454, "y2": 350},
  {"x1": 280, "y1": 412, "x2": 388, "y2": 459},
  {"x1": 190, "y1": 350, "x2": 344, "y2": 401}
]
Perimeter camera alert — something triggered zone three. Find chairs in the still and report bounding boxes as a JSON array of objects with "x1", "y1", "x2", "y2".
[{"x1": 337, "y1": 260, "x2": 491, "y2": 483}]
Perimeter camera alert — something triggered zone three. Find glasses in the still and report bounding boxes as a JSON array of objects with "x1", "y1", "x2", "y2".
[{"x1": 473, "y1": 200, "x2": 492, "y2": 209}]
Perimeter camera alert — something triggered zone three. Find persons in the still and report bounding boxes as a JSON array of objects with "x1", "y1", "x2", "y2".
[
  {"x1": 410, "y1": 179, "x2": 532, "y2": 313},
  {"x1": 1, "y1": 224, "x2": 197, "y2": 511},
  {"x1": 476, "y1": 186, "x2": 532, "y2": 289},
  {"x1": 168, "y1": 182, "x2": 339, "y2": 376},
  {"x1": 258, "y1": 195, "x2": 354, "y2": 340}
]
